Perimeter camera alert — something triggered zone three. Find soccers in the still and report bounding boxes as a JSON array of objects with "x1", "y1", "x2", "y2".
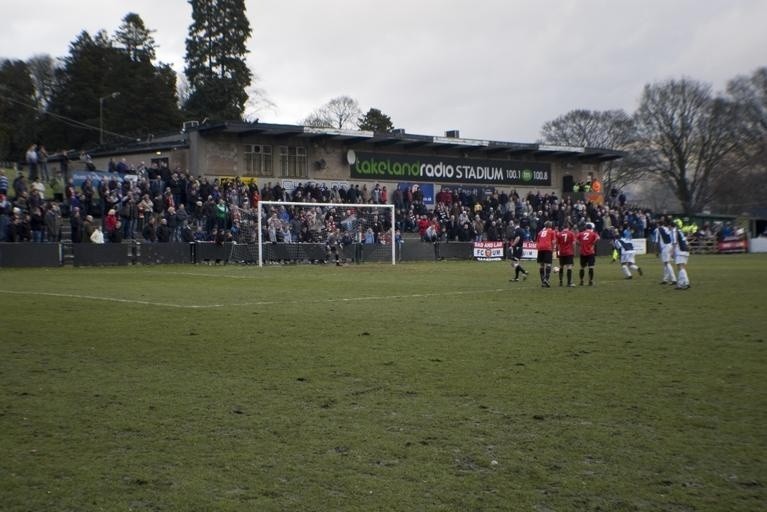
[{"x1": 554, "y1": 266, "x2": 560, "y2": 273}]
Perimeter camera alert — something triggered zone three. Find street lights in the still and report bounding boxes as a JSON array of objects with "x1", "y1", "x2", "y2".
[{"x1": 100, "y1": 91, "x2": 121, "y2": 144}]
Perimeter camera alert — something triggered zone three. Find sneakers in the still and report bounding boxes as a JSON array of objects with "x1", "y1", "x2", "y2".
[
  {"x1": 624, "y1": 276, "x2": 632, "y2": 279},
  {"x1": 510, "y1": 278, "x2": 519, "y2": 282},
  {"x1": 524, "y1": 271, "x2": 528, "y2": 279},
  {"x1": 658, "y1": 281, "x2": 689, "y2": 289},
  {"x1": 638, "y1": 268, "x2": 641, "y2": 275},
  {"x1": 542, "y1": 279, "x2": 593, "y2": 287}
]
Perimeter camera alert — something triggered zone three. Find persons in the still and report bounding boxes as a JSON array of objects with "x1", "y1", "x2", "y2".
[
  {"x1": 1, "y1": 144, "x2": 393, "y2": 267},
  {"x1": 392, "y1": 176, "x2": 736, "y2": 291}
]
